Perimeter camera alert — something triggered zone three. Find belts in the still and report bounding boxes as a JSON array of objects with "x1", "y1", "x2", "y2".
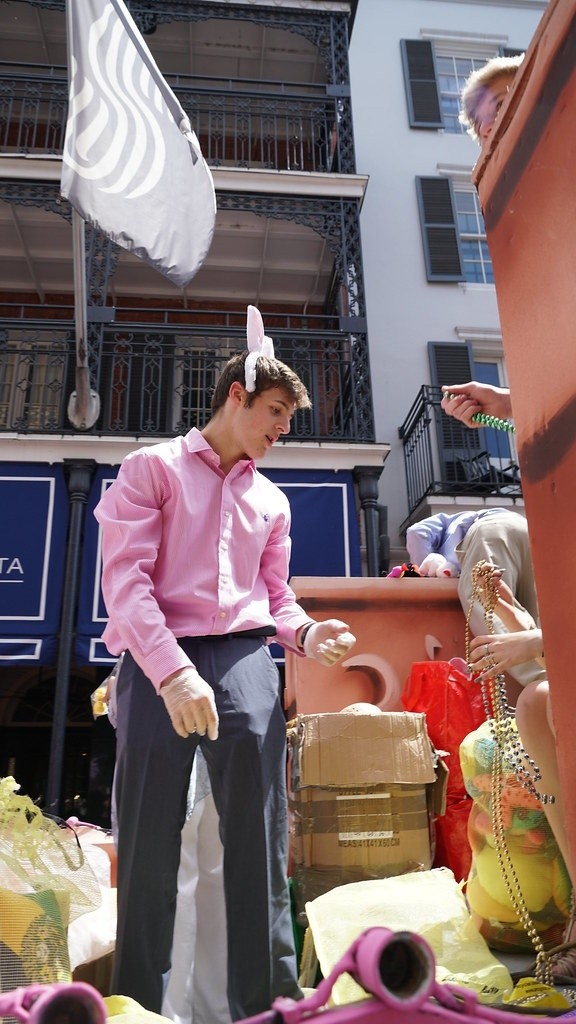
[{"x1": 193, "y1": 627, "x2": 276, "y2": 638}]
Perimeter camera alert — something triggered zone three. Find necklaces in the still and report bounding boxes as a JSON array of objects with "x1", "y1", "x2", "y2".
[
  {"x1": 465, "y1": 560, "x2": 557, "y2": 985},
  {"x1": 442, "y1": 391, "x2": 515, "y2": 433}
]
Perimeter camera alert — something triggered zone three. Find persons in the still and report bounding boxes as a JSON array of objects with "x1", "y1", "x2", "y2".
[
  {"x1": 440, "y1": 51, "x2": 530, "y2": 428},
  {"x1": 406, "y1": 508, "x2": 546, "y2": 687},
  {"x1": 94, "y1": 351, "x2": 355, "y2": 1024},
  {"x1": 465, "y1": 560, "x2": 575, "y2": 982}
]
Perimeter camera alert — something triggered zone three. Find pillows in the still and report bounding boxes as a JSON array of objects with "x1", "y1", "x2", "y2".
[{"x1": 0, "y1": 889, "x2": 72, "y2": 1024}]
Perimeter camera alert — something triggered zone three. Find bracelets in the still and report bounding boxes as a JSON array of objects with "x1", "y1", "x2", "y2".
[{"x1": 301, "y1": 622, "x2": 315, "y2": 644}]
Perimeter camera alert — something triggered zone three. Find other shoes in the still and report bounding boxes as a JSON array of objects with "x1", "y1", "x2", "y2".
[{"x1": 552, "y1": 914, "x2": 576, "y2": 985}]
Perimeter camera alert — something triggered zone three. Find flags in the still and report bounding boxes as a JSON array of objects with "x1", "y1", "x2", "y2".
[{"x1": 59, "y1": 0, "x2": 216, "y2": 288}]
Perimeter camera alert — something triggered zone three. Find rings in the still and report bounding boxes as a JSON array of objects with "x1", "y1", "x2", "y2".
[
  {"x1": 490, "y1": 655, "x2": 495, "y2": 664},
  {"x1": 483, "y1": 645, "x2": 490, "y2": 654}
]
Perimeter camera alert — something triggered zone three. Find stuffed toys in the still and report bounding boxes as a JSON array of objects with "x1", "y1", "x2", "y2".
[{"x1": 419, "y1": 553, "x2": 458, "y2": 577}]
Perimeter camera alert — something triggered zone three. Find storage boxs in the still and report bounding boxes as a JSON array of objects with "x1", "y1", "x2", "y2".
[{"x1": 292, "y1": 712, "x2": 453, "y2": 927}]
